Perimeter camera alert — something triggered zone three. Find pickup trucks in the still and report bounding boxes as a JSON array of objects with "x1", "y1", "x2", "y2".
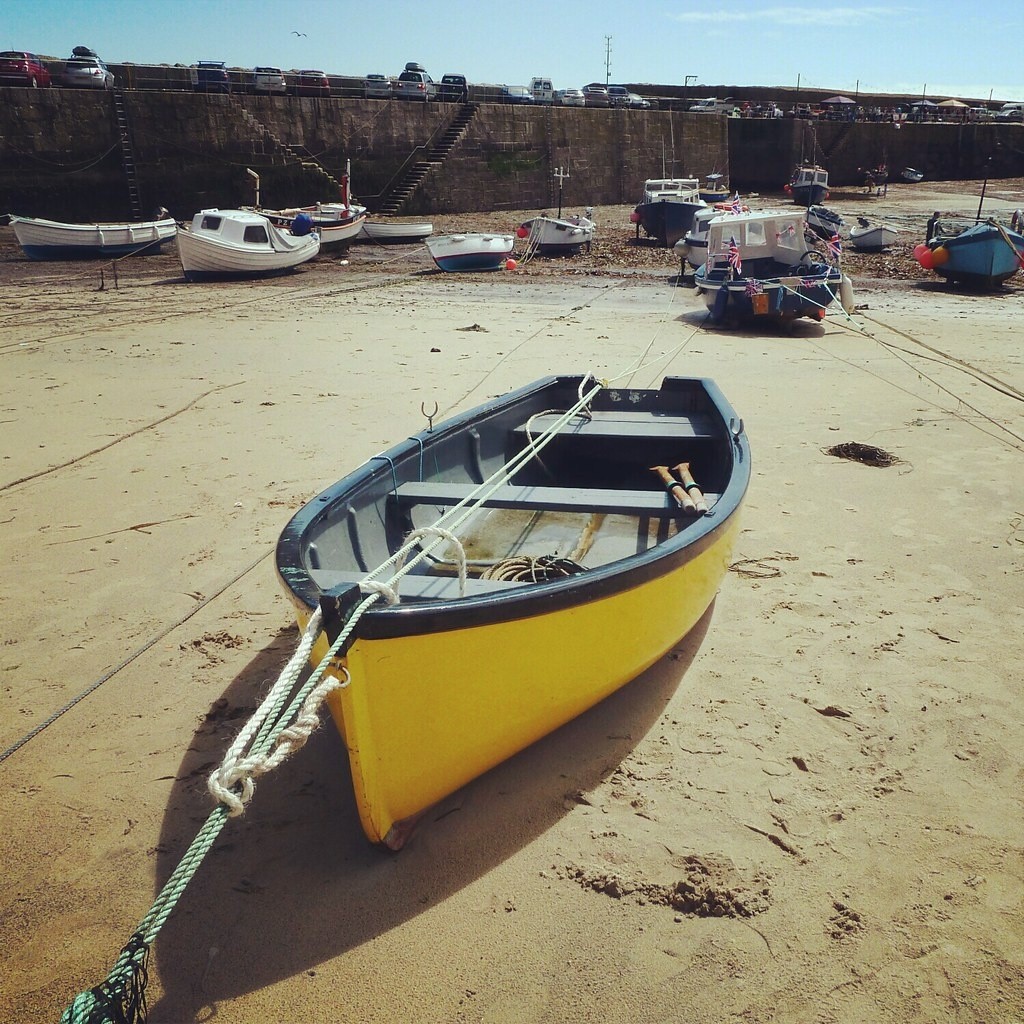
[{"x1": 689, "y1": 98, "x2": 734, "y2": 115}]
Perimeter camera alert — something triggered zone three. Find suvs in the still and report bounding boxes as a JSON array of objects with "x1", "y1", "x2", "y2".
[{"x1": 528, "y1": 77, "x2": 554, "y2": 106}]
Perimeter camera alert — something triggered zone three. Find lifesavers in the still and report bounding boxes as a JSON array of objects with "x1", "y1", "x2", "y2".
[{"x1": 1010, "y1": 210, "x2": 1024, "y2": 235}]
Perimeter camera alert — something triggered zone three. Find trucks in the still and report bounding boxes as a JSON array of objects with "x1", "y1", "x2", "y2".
[{"x1": 1002, "y1": 103, "x2": 1024, "y2": 115}]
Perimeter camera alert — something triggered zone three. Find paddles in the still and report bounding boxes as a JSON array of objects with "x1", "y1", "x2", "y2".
[
  {"x1": 670, "y1": 448, "x2": 708, "y2": 517},
  {"x1": 650, "y1": 466, "x2": 696, "y2": 514}
]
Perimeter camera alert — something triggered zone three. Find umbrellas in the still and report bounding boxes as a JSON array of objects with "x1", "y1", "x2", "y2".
[
  {"x1": 820, "y1": 95, "x2": 856, "y2": 103},
  {"x1": 937, "y1": 99, "x2": 969, "y2": 108},
  {"x1": 910, "y1": 100, "x2": 937, "y2": 106}
]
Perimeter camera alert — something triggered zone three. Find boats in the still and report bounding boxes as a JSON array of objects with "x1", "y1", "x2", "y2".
[
  {"x1": 783, "y1": 129, "x2": 830, "y2": 207},
  {"x1": 240, "y1": 159, "x2": 366, "y2": 251},
  {"x1": 175, "y1": 208, "x2": 322, "y2": 281},
  {"x1": 849, "y1": 217, "x2": 897, "y2": 253},
  {"x1": 275, "y1": 373, "x2": 753, "y2": 853},
  {"x1": 517, "y1": 167, "x2": 597, "y2": 258},
  {"x1": 672, "y1": 188, "x2": 767, "y2": 270},
  {"x1": 7, "y1": 207, "x2": 178, "y2": 260},
  {"x1": 355, "y1": 221, "x2": 434, "y2": 243},
  {"x1": 420, "y1": 233, "x2": 514, "y2": 272},
  {"x1": 694, "y1": 209, "x2": 842, "y2": 327},
  {"x1": 913, "y1": 178, "x2": 1024, "y2": 292},
  {"x1": 698, "y1": 173, "x2": 730, "y2": 203},
  {"x1": 634, "y1": 135, "x2": 708, "y2": 247}
]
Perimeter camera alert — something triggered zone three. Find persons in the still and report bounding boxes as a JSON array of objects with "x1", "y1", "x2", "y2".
[
  {"x1": 770, "y1": 101, "x2": 777, "y2": 118},
  {"x1": 925, "y1": 210, "x2": 946, "y2": 245},
  {"x1": 877, "y1": 184, "x2": 887, "y2": 199},
  {"x1": 805, "y1": 104, "x2": 811, "y2": 114},
  {"x1": 827, "y1": 104, "x2": 903, "y2": 123},
  {"x1": 957, "y1": 110, "x2": 975, "y2": 124},
  {"x1": 743, "y1": 103, "x2": 762, "y2": 117}
]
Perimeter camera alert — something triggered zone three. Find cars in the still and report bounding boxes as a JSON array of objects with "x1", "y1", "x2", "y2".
[
  {"x1": 497, "y1": 85, "x2": 651, "y2": 110},
  {"x1": 970, "y1": 107, "x2": 1024, "y2": 123},
  {"x1": 0, "y1": 45, "x2": 469, "y2": 102}
]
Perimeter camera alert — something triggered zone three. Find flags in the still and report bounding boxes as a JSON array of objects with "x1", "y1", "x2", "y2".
[
  {"x1": 745, "y1": 280, "x2": 763, "y2": 298},
  {"x1": 728, "y1": 235, "x2": 742, "y2": 276},
  {"x1": 828, "y1": 228, "x2": 842, "y2": 258},
  {"x1": 731, "y1": 191, "x2": 741, "y2": 216}
]
